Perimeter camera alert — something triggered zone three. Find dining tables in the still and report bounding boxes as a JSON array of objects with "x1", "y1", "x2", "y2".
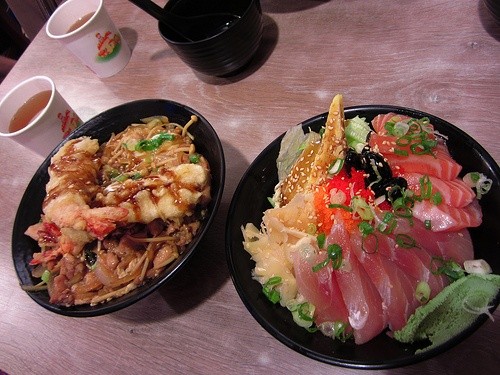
[{"x1": 0, "y1": 1, "x2": 500, "y2": 375}]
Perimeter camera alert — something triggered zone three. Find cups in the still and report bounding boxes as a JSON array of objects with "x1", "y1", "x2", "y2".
[
  {"x1": 46, "y1": 0, "x2": 131, "y2": 79},
  {"x1": 158, "y1": 0, "x2": 264, "y2": 77},
  {"x1": 0, "y1": 76, "x2": 86, "y2": 161}
]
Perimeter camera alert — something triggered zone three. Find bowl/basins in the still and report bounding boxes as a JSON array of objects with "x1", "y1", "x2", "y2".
[
  {"x1": 13, "y1": 98, "x2": 228, "y2": 317},
  {"x1": 226, "y1": 104, "x2": 500, "y2": 371}
]
